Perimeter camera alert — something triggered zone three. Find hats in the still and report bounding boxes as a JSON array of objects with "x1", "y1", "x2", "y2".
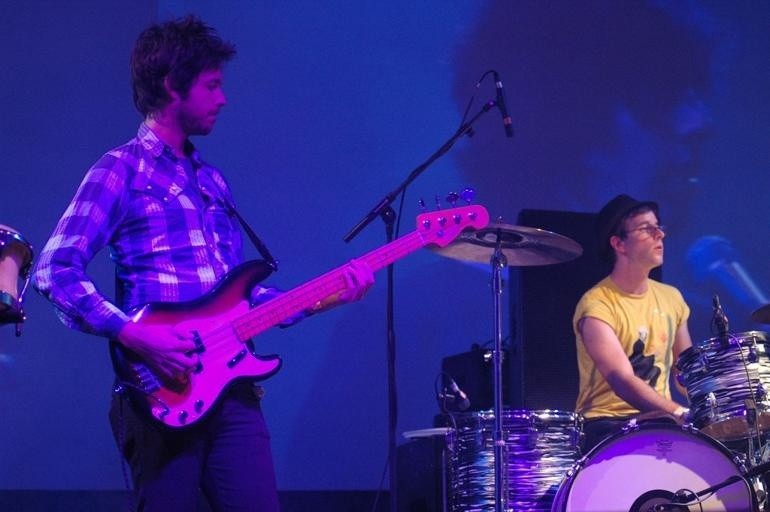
[{"x1": 594, "y1": 193, "x2": 659, "y2": 251}]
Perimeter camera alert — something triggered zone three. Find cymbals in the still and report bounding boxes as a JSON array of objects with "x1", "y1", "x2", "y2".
[
  {"x1": 751, "y1": 304, "x2": 770, "y2": 323},
  {"x1": 425, "y1": 223, "x2": 583, "y2": 267}
]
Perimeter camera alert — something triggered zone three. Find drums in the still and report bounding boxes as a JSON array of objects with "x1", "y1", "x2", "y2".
[
  {"x1": 445, "y1": 408, "x2": 584, "y2": 511},
  {"x1": 551, "y1": 417, "x2": 760, "y2": 512},
  {"x1": 0, "y1": 225, "x2": 34, "y2": 325},
  {"x1": 675, "y1": 331, "x2": 770, "y2": 442}
]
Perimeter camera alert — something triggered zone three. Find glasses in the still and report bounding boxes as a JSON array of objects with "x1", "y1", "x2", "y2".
[{"x1": 619, "y1": 224, "x2": 667, "y2": 237}]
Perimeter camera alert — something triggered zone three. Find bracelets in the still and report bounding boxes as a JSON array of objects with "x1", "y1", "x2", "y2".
[{"x1": 672, "y1": 405, "x2": 688, "y2": 419}]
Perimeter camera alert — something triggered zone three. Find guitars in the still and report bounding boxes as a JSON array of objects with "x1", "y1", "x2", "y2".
[{"x1": 109, "y1": 189, "x2": 490, "y2": 431}]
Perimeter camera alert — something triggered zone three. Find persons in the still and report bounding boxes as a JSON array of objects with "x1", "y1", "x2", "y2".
[
  {"x1": 572, "y1": 194, "x2": 695, "y2": 457},
  {"x1": 28, "y1": 16, "x2": 375, "y2": 512}
]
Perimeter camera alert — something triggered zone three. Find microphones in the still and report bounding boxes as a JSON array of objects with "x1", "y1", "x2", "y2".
[
  {"x1": 713, "y1": 295, "x2": 729, "y2": 332},
  {"x1": 493, "y1": 72, "x2": 515, "y2": 137},
  {"x1": 445, "y1": 373, "x2": 471, "y2": 411}
]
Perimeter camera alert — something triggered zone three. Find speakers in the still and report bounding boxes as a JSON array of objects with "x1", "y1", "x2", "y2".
[{"x1": 507, "y1": 209, "x2": 662, "y2": 414}]
